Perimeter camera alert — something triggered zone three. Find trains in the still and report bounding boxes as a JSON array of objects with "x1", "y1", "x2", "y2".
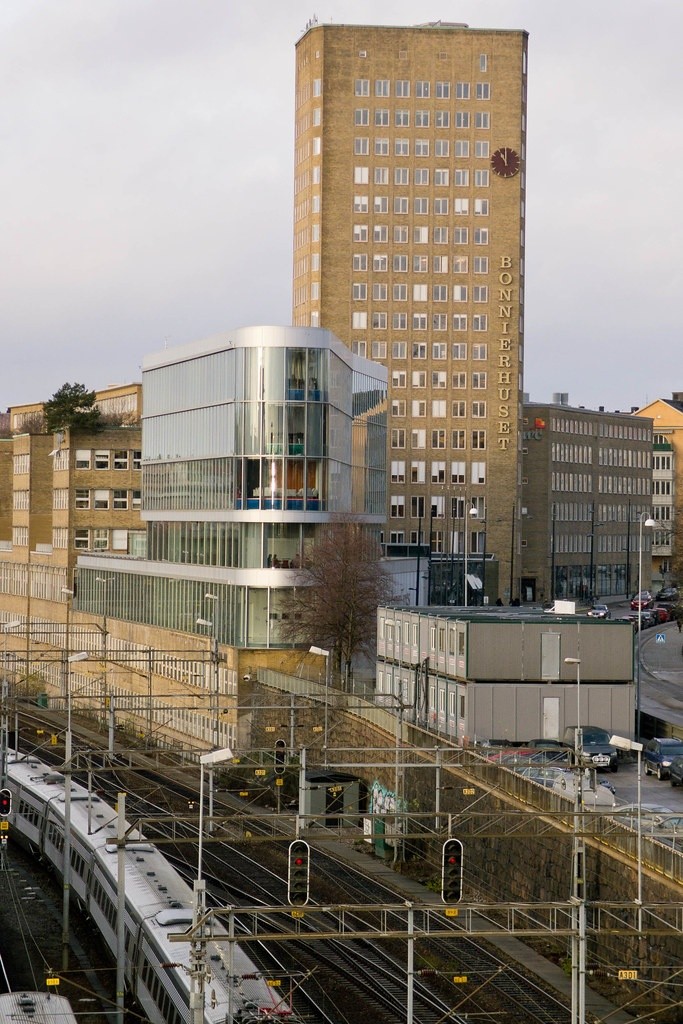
[
  {"x1": 0, "y1": 992, "x2": 77, "y2": 1024},
  {"x1": 4, "y1": 748, "x2": 301, "y2": 1024}
]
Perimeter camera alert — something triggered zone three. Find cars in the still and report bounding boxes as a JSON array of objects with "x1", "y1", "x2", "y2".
[
  {"x1": 631, "y1": 590, "x2": 654, "y2": 611},
  {"x1": 587, "y1": 604, "x2": 612, "y2": 621},
  {"x1": 656, "y1": 587, "x2": 679, "y2": 602},
  {"x1": 608, "y1": 802, "x2": 675, "y2": 833},
  {"x1": 646, "y1": 810, "x2": 682, "y2": 847},
  {"x1": 484, "y1": 739, "x2": 617, "y2": 803},
  {"x1": 615, "y1": 604, "x2": 682, "y2": 634},
  {"x1": 668, "y1": 755, "x2": 683, "y2": 788}
]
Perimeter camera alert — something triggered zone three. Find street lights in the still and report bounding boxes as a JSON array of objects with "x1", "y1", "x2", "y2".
[
  {"x1": 637, "y1": 514, "x2": 655, "y2": 744},
  {"x1": 287, "y1": 839, "x2": 310, "y2": 906},
  {"x1": 565, "y1": 657, "x2": 582, "y2": 765},
  {"x1": 465, "y1": 501, "x2": 479, "y2": 607},
  {"x1": 441, "y1": 838, "x2": 464, "y2": 905}
]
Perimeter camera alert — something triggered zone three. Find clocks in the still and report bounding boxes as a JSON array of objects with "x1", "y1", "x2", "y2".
[{"x1": 490, "y1": 146, "x2": 522, "y2": 177}]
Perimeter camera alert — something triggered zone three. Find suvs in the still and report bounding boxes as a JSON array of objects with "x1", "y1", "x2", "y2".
[
  {"x1": 562, "y1": 725, "x2": 618, "y2": 774},
  {"x1": 644, "y1": 736, "x2": 683, "y2": 781}
]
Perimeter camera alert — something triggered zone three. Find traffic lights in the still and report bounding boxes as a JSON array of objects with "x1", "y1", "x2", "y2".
[
  {"x1": 0, "y1": 789, "x2": 13, "y2": 817},
  {"x1": 274, "y1": 739, "x2": 286, "y2": 774}
]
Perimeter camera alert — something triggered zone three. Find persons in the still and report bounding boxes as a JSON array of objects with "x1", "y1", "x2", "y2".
[
  {"x1": 494, "y1": 594, "x2": 552, "y2": 611},
  {"x1": 266, "y1": 552, "x2": 311, "y2": 570}
]
